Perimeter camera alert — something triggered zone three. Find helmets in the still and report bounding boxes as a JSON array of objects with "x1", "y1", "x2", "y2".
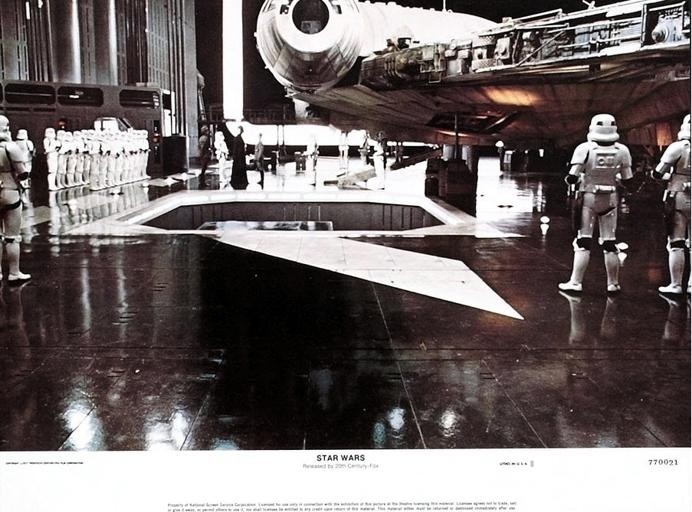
[
  {"x1": 0, "y1": 116, "x2": 12, "y2": 141},
  {"x1": 44, "y1": 127, "x2": 149, "y2": 141},
  {"x1": 17, "y1": 129, "x2": 29, "y2": 140},
  {"x1": 587, "y1": 113, "x2": 620, "y2": 143},
  {"x1": 679, "y1": 113, "x2": 690, "y2": 139}
]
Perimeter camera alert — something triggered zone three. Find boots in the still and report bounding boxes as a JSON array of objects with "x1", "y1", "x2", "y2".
[
  {"x1": 558, "y1": 249, "x2": 591, "y2": 292},
  {"x1": 658, "y1": 247, "x2": 685, "y2": 295},
  {"x1": 0, "y1": 234, "x2": 31, "y2": 280},
  {"x1": 48, "y1": 164, "x2": 152, "y2": 191},
  {"x1": 602, "y1": 250, "x2": 622, "y2": 292}
]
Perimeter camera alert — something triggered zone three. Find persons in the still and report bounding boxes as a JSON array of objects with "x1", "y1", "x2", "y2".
[
  {"x1": 199, "y1": 125, "x2": 214, "y2": 178},
  {"x1": 555, "y1": 113, "x2": 635, "y2": 296},
  {"x1": 649, "y1": 113, "x2": 690, "y2": 297},
  {"x1": 0, "y1": 115, "x2": 36, "y2": 282},
  {"x1": 636, "y1": 293, "x2": 690, "y2": 446},
  {"x1": 21, "y1": 198, "x2": 41, "y2": 255},
  {"x1": 308, "y1": 134, "x2": 318, "y2": 186},
  {"x1": 372, "y1": 131, "x2": 388, "y2": 191},
  {"x1": 232, "y1": 125, "x2": 250, "y2": 190},
  {"x1": 553, "y1": 288, "x2": 621, "y2": 447},
  {"x1": 1, "y1": 282, "x2": 38, "y2": 450},
  {"x1": 40, "y1": 123, "x2": 153, "y2": 193},
  {"x1": 46, "y1": 192, "x2": 157, "y2": 264},
  {"x1": 253, "y1": 133, "x2": 265, "y2": 189},
  {"x1": 15, "y1": 129, "x2": 38, "y2": 205}
]
